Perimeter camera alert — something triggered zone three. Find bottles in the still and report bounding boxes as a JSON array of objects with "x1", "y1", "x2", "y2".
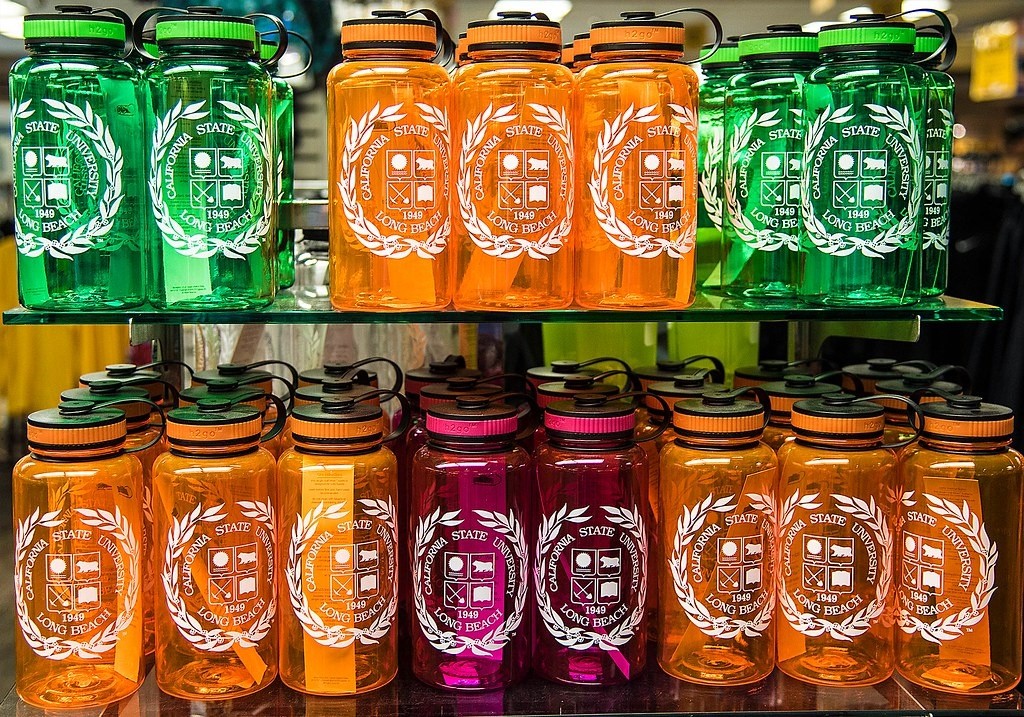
[
  {"x1": 894, "y1": 386, "x2": 1024, "y2": 696},
  {"x1": 275, "y1": 388, "x2": 414, "y2": 699},
  {"x1": 774, "y1": 391, "x2": 925, "y2": 687},
  {"x1": 410, "y1": 390, "x2": 541, "y2": 696},
  {"x1": 57, "y1": 375, "x2": 179, "y2": 658},
  {"x1": 152, "y1": 381, "x2": 287, "y2": 700},
  {"x1": 12, "y1": 391, "x2": 163, "y2": 710},
  {"x1": 657, "y1": 386, "x2": 773, "y2": 689},
  {"x1": 13, "y1": 0, "x2": 957, "y2": 311},
  {"x1": 535, "y1": 391, "x2": 673, "y2": 690},
  {"x1": 59, "y1": 320, "x2": 974, "y2": 458}
]
